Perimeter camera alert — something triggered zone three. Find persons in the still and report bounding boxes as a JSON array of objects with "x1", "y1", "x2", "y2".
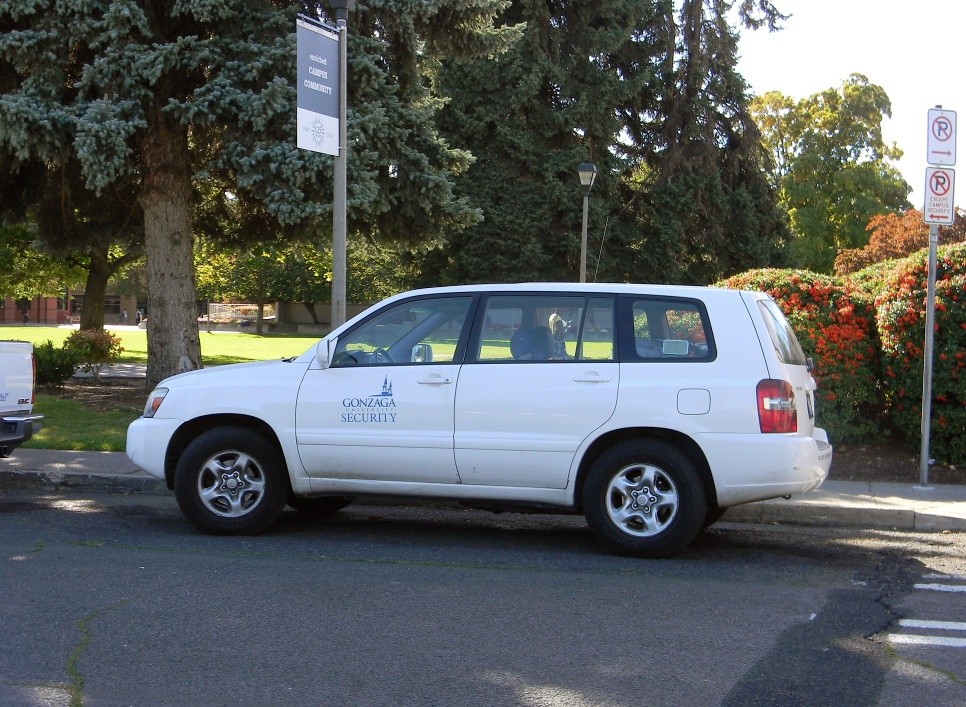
[
  {"x1": 21, "y1": 308, "x2": 29, "y2": 325},
  {"x1": 123, "y1": 308, "x2": 127, "y2": 325},
  {"x1": 549, "y1": 308, "x2": 571, "y2": 356},
  {"x1": 136, "y1": 309, "x2": 141, "y2": 325}
]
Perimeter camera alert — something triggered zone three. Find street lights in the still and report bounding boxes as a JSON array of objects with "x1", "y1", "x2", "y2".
[{"x1": 577, "y1": 164, "x2": 597, "y2": 342}]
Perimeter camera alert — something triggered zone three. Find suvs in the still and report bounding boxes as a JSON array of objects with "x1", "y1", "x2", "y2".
[{"x1": 126, "y1": 281, "x2": 833, "y2": 559}]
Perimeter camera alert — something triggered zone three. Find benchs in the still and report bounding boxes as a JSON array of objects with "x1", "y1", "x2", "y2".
[{"x1": 69, "y1": 316, "x2": 80, "y2": 324}]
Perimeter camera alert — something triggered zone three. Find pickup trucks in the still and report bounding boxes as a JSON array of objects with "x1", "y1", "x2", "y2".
[{"x1": 0, "y1": 340, "x2": 45, "y2": 459}]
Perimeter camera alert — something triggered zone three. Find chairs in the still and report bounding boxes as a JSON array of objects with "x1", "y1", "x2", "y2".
[{"x1": 530, "y1": 327, "x2": 555, "y2": 360}]
